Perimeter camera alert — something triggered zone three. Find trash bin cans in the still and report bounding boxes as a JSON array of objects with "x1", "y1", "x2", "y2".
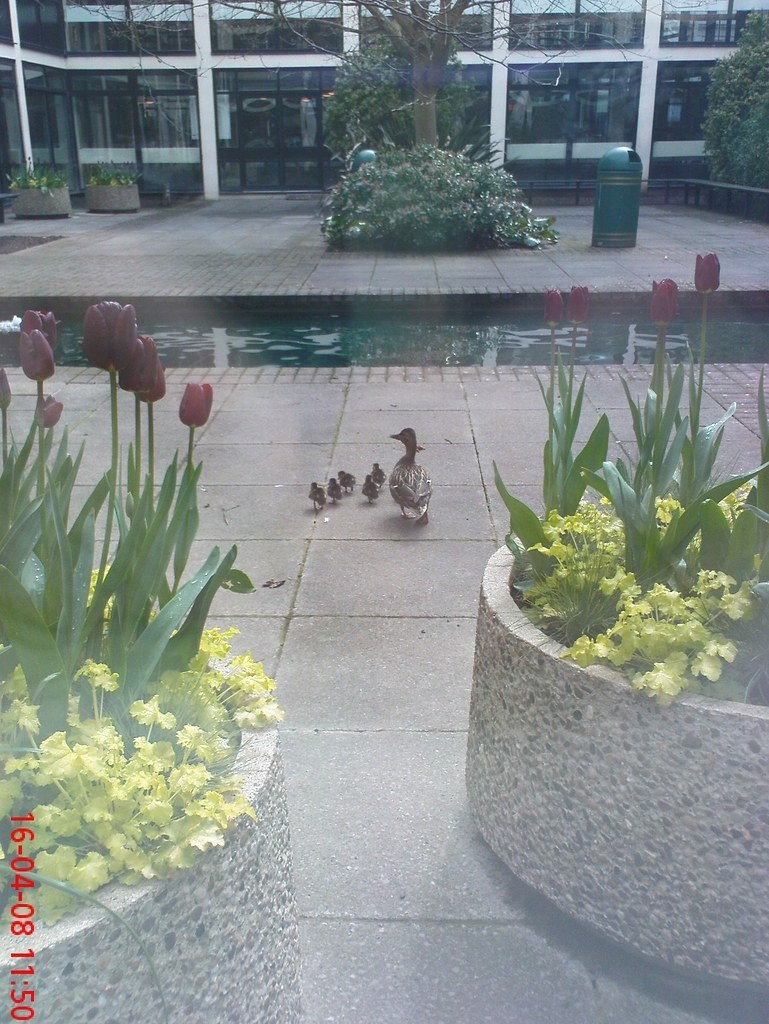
[{"x1": 589, "y1": 146, "x2": 644, "y2": 249}]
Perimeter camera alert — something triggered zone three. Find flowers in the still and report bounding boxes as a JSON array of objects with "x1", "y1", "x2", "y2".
[
  {"x1": 5, "y1": 157, "x2": 70, "y2": 198},
  {"x1": 87, "y1": 160, "x2": 142, "y2": 189},
  {"x1": 0, "y1": 299, "x2": 282, "y2": 924},
  {"x1": 491, "y1": 253, "x2": 769, "y2": 701}
]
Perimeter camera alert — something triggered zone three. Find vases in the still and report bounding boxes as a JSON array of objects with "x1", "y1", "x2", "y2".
[
  {"x1": 84, "y1": 183, "x2": 140, "y2": 213},
  {"x1": 0, "y1": 647, "x2": 299, "y2": 1023},
  {"x1": 9, "y1": 187, "x2": 70, "y2": 218},
  {"x1": 464, "y1": 538, "x2": 768, "y2": 986}
]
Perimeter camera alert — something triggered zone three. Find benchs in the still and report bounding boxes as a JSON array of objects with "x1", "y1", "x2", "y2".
[{"x1": 512, "y1": 178, "x2": 768, "y2": 224}]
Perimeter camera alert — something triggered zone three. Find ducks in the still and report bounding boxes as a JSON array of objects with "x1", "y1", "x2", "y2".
[
  {"x1": 388, "y1": 428, "x2": 433, "y2": 526},
  {"x1": 308, "y1": 470, "x2": 356, "y2": 514},
  {"x1": 361, "y1": 463, "x2": 387, "y2": 503}
]
